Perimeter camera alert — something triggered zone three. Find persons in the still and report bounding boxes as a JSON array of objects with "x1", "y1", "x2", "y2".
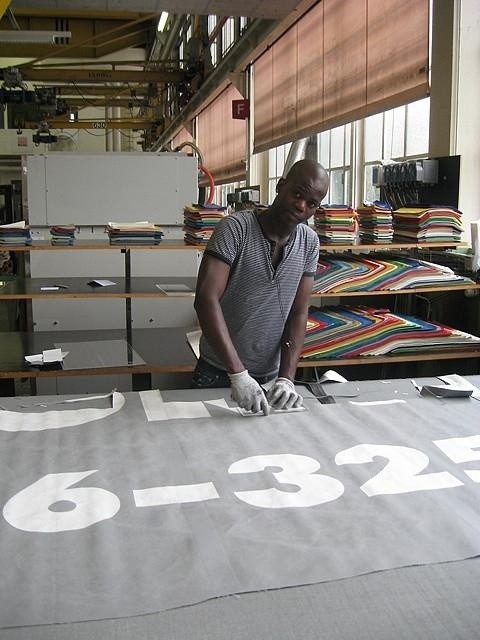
[{"x1": 193, "y1": 157, "x2": 331, "y2": 417}]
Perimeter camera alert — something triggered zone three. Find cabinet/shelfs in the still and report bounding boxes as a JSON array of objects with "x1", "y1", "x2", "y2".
[{"x1": 0, "y1": 239, "x2": 480, "y2": 397}]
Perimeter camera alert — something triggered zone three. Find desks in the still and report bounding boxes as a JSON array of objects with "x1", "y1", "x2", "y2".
[{"x1": 0, "y1": 374, "x2": 480, "y2": 640}]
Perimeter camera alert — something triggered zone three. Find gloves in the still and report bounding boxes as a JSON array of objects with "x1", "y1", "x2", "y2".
[
  {"x1": 266, "y1": 377, "x2": 305, "y2": 410},
  {"x1": 227, "y1": 370, "x2": 271, "y2": 417}
]
